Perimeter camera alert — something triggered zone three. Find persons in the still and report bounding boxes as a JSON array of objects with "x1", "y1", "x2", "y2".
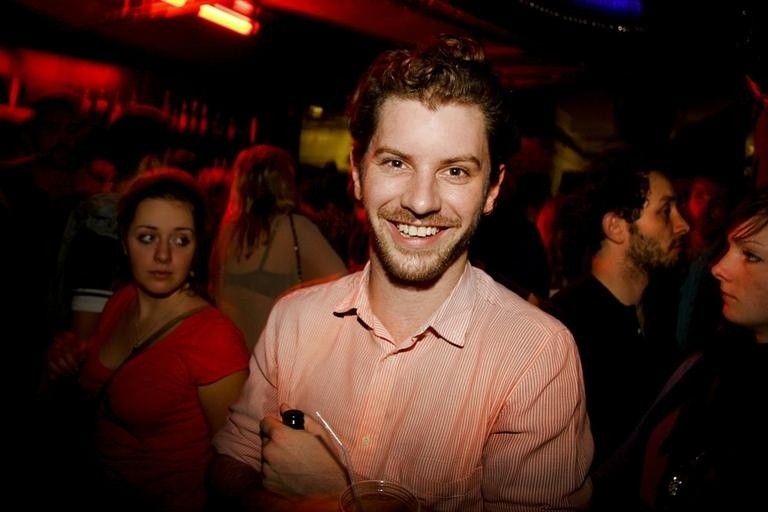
[
  {"x1": 474, "y1": 126, "x2": 768, "y2": 512},
  {"x1": 202, "y1": 31, "x2": 599, "y2": 509},
  {"x1": 0, "y1": 96, "x2": 348, "y2": 512}
]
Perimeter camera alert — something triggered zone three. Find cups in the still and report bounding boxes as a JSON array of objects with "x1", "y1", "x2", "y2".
[{"x1": 339, "y1": 481, "x2": 421, "y2": 512}]
